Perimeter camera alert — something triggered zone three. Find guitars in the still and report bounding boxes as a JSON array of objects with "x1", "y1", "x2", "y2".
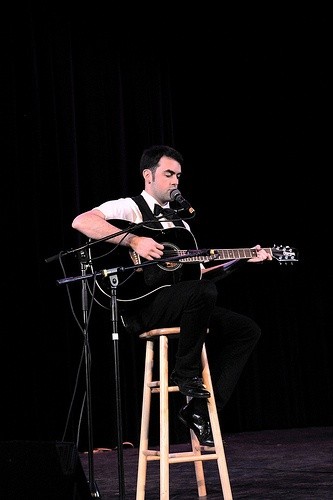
[{"x1": 84, "y1": 219, "x2": 300, "y2": 312}]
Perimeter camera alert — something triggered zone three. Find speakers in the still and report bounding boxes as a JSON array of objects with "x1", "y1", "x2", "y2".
[{"x1": 0, "y1": 440, "x2": 93, "y2": 500}]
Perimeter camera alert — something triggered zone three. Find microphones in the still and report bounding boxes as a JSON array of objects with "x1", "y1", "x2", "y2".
[
  {"x1": 207, "y1": 249, "x2": 218, "y2": 255},
  {"x1": 170, "y1": 189, "x2": 196, "y2": 214}
]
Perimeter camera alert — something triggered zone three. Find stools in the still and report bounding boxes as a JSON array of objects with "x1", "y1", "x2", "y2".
[{"x1": 137, "y1": 324, "x2": 232, "y2": 500}]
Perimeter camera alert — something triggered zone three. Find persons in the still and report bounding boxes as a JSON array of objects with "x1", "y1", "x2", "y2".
[{"x1": 72, "y1": 142, "x2": 273, "y2": 446}]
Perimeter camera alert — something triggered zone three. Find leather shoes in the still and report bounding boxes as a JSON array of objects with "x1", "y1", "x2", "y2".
[
  {"x1": 170, "y1": 370, "x2": 210, "y2": 398},
  {"x1": 178, "y1": 405, "x2": 224, "y2": 447}
]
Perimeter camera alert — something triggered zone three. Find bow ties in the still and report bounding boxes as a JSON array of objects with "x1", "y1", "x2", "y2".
[{"x1": 153, "y1": 204, "x2": 174, "y2": 219}]
